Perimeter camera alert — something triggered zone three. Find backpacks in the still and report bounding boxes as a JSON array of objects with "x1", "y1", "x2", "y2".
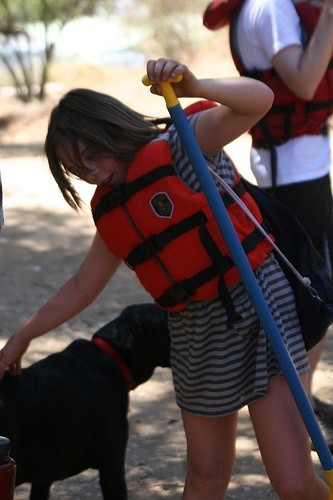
[{"x1": 151, "y1": 118, "x2": 333, "y2": 350}]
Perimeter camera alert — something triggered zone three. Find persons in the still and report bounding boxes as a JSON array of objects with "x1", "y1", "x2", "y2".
[
  {"x1": 229, "y1": 0, "x2": 333, "y2": 456},
  {"x1": 0, "y1": 58, "x2": 333, "y2": 500}
]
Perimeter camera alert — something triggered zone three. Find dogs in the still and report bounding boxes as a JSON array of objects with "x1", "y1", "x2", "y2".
[{"x1": 0, "y1": 302, "x2": 171, "y2": 500}]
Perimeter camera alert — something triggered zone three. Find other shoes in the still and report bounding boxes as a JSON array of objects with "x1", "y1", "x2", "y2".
[{"x1": 313, "y1": 396, "x2": 333, "y2": 429}]
